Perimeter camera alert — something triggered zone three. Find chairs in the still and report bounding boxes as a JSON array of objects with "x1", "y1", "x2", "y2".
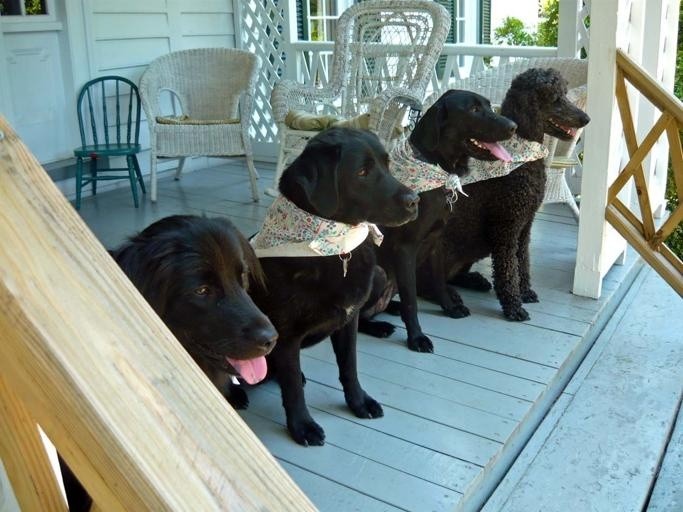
[
  {"x1": 137, "y1": 47, "x2": 264, "y2": 202},
  {"x1": 262, "y1": 0, "x2": 451, "y2": 199},
  {"x1": 418, "y1": 54, "x2": 587, "y2": 226},
  {"x1": 74, "y1": 75, "x2": 146, "y2": 211}
]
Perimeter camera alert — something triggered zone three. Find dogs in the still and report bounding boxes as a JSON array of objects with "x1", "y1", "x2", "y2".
[
  {"x1": 357, "y1": 89, "x2": 519, "y2": 353},
  {"x1": 417, "y1": 66, "x2": 592, "y2": 324},
  {"x1": 57, "y1": 214, "x2": 281, "y2": 512},
  {"x1": 246, "y1": 124, "x2": 422, "y2": 448}
]
